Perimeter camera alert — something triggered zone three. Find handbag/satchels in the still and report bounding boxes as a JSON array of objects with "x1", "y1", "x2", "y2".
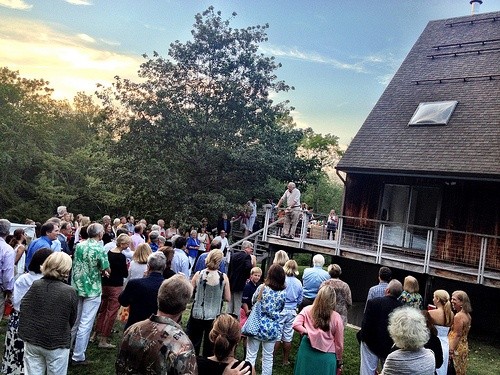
[{"x1": 240, "y1": 284, "x2": 265, "y2": 337}]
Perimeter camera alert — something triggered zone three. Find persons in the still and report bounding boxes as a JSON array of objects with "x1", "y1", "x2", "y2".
[
  {"x1": 17, "y1": 251, "x2": 79, "y2": 375},
  {"x1": 196, "y1": 312, "x2": 253, "y2": 375},
  {"x1": 116, "y1": 274, "x2": 198, "y2": 375},
  {"x1": 0, "y1": 218, "x2": 16, "y2": 322},
  {"x1": 68, "y1": 222, "x2": 111, "y2": 366},
  {"x1": 290, "y1": 285, "x2": 344, "y2": 375},
  {"x1": 243, "y1": 264, "x2": 287, "y2": 374},
  {"x1": 358, "y1": 265, "x2": 471, "y2": 375},
  {"x1": 222, "y1": 194, "x2": 339, "y2": 244},
  {"x1": 376, "y1": 306, "x2": 436, "y2": 375},
  {"x1": 5, "y1": 205, "x2": 353, "y2": 365},
  {"x1": 274, "y1": 179, "x2": 301, "y2": 237},
  {"x1": 2, "y1": 246, "x2": 54, "y2": 375}
]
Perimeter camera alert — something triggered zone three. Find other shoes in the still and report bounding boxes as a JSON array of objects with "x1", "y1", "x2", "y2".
[
  {"x1": 283, "y1": 234, "x2": 288, "y2": 238},
  {"x1": 98, "y1": 342, "x2": 116, "y2": 349},
  {"x1": 288, "y1": 234, "x2": 294, "y2": 239},
  {"x1": 89, "y1": 336, "x2": 100, "y2": 344},
  {"x1": 70, "y1": 360, "x2": 94, "y2": 367}
]
